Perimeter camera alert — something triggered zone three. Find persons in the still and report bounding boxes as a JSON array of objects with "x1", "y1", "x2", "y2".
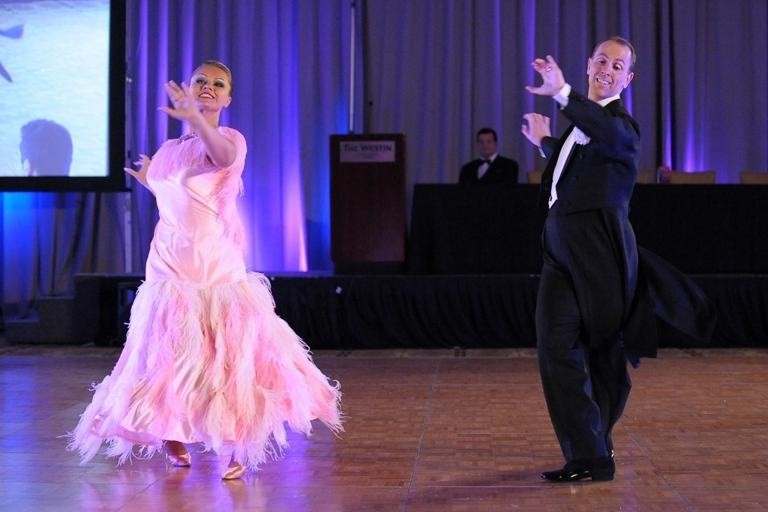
[
  {"x1": 61, "y1": 61, "x2": 343, "y2": 480},
  {"x1": 520, "y1": 36, "x2": 704, "y2": 484},
  {"x1": 459, "y1": 127, "x2": 519, "y2": 185}
]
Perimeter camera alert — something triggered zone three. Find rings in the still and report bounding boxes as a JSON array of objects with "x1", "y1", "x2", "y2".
[{"x1": 546, "y1": 68, "x2": 551, "y2": 72}]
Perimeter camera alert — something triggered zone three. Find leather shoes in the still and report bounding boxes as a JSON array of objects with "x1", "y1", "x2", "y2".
[
  {"x1": 163, "y1": 445, "x2": 192, "y2": 467},
  {"x1": 606, "y1": 432, "x2": 615, "y2": 459},
  {"x1": 542, "y1": 462, "x2": 616, "y2": 484},
  {"x1": 221, "y1": 456, "x2": 247, "y2": 481}
]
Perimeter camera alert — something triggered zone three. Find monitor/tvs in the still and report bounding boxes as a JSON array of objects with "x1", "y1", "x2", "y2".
[{"x1": 0, "y1": 0, "x2": 126, "y2": 193}]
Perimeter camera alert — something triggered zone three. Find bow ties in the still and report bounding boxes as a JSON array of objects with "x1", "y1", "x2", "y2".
[{"x1": 480, "y1": 159, "x2": 492, "y2": 167}]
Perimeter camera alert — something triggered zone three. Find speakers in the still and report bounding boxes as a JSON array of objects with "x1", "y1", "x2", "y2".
[{"x1": 329, "y1": 133, "x2": 408, "y2": 278}]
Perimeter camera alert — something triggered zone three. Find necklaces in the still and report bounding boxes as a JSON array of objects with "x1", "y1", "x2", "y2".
[{"x1": 177, "y1": 130, "x2": 200, "y2": 143}]
